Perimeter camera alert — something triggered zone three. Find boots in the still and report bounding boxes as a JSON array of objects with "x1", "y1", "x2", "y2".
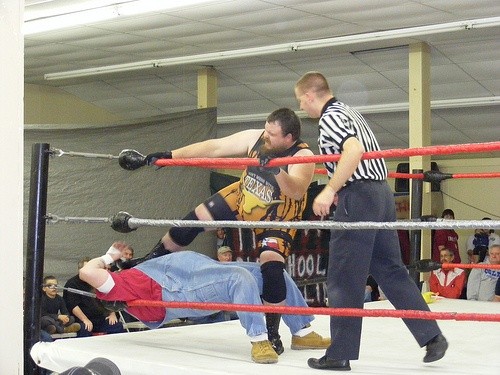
[
  {"x1": 264, "y1": 312, "x2": 284, "y2": 356},
  {"x1": 118, "y1": 238, "x2": 172, "y2": 270}
]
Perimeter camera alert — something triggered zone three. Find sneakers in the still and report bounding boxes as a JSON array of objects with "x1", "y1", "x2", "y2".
[
  {"x1": 250, "y1": 340, "x2": 278, "y2": 364},
  {"x1": 291, "y1": 331, "x2": 332, "y2": 351}
]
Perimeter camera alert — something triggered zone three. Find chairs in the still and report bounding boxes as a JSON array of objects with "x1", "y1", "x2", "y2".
[{"x1": 51, "y1": 311, "x2": 180, "y2": 340}]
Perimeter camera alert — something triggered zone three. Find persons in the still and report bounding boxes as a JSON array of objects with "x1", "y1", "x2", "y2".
[
  {"x1": 466, "y1": 244, "x2": 500, "y2": 301},
  {"x1": 121, "y1": 247, "x2": 133, "y2": 262},
  {"x1": 429, "y1": 248, "x2": 467, "y2": 299},
  {"x1": 295, "y1": 72, "x2": 449, "y2": 371},
  {"x1": 64, "y1": 257, "x2": 127, "y2": 338},
  {"x1": 217, "y1": 246, "x2": 232, "y2": 261},
  {"x1": 40, "y1": 276, "x2": 81, "y2": 342},
  {"x1": 79, "y1": 241, "x2": 330, "y2": 363},
  {"x1": 467, "y1": 218, "x2": 500, "y2": 264},
  {"x1": 119, "y1": 108, "x2": 315, "y2": 354},
  {"x1": 432, "y1": 209, "x2": 461, "y2": 264}
]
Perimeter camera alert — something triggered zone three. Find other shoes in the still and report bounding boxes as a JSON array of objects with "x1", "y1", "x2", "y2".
[
  {"x1": 64, "y1": 323, "x2": 80, "y2": 333},
  {"x1": 46, "y1": 325, "x2": 57, "y2": 334}
]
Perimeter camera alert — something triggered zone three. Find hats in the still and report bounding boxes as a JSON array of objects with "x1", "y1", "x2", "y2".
[{"x1": 58, "y1": 357, "x2": 122, "y2": 375}]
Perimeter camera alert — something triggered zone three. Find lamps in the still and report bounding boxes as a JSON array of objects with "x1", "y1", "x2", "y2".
[
  {"x1": 217, "y1": 96, "x2": 500, "y2": 123},
  {"x1": 44, "y1": 17, "x2": 500, "y2": 81}
]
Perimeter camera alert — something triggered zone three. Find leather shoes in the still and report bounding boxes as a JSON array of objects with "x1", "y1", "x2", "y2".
[
  {"x1": 423, "y1": 334, "x2": 448, "y2": 362},
  {"x1": 307, "y1": 355, "x2": 351, "y2": 371}
]
